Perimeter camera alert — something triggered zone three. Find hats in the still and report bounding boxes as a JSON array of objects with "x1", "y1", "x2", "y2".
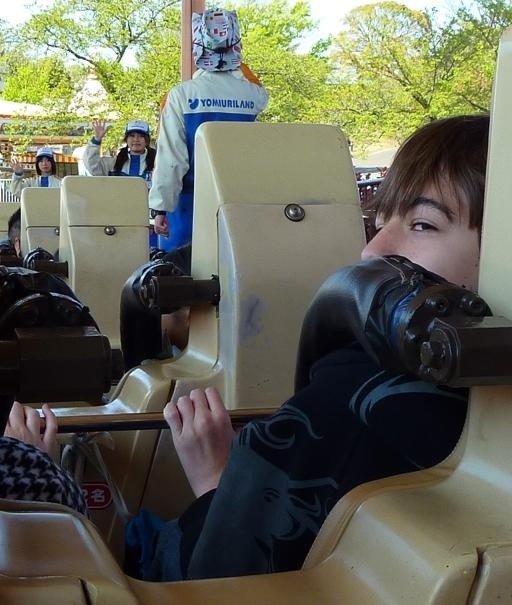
[
  {"x1": 35, "y1": 147, "x2": 53, "y2": 159},
  {"x1": 190, "y1": 8, "x2": 243, "y2": 74},
  {"x1": 124, "y1": 119, "x2": 150, "y2": 136}
]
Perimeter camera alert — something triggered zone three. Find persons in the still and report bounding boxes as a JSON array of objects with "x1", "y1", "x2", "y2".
[
  {"x1": 82, "y1": 118, "x2": 158, "y2": 251},
  {"x1": 9, "y1": 148, "x2": 62, "y2": 200},
  {"x1": 0, "y1": 355, "x2": 90, "y2": 529},
  {"x1": 163, "y1": 113, "x2": 490, "y2": 575},
  {"x1": 149, "y1": 7, "x2": 269, "y2": 353},
  {"x1": 8, "y1": 207, "x2": 33, "y2": 260}
]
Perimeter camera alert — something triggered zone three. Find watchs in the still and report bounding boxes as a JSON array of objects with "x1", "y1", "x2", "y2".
[{"x1": 149, "y1": 209, "x2": 167, "y2": 218}]
